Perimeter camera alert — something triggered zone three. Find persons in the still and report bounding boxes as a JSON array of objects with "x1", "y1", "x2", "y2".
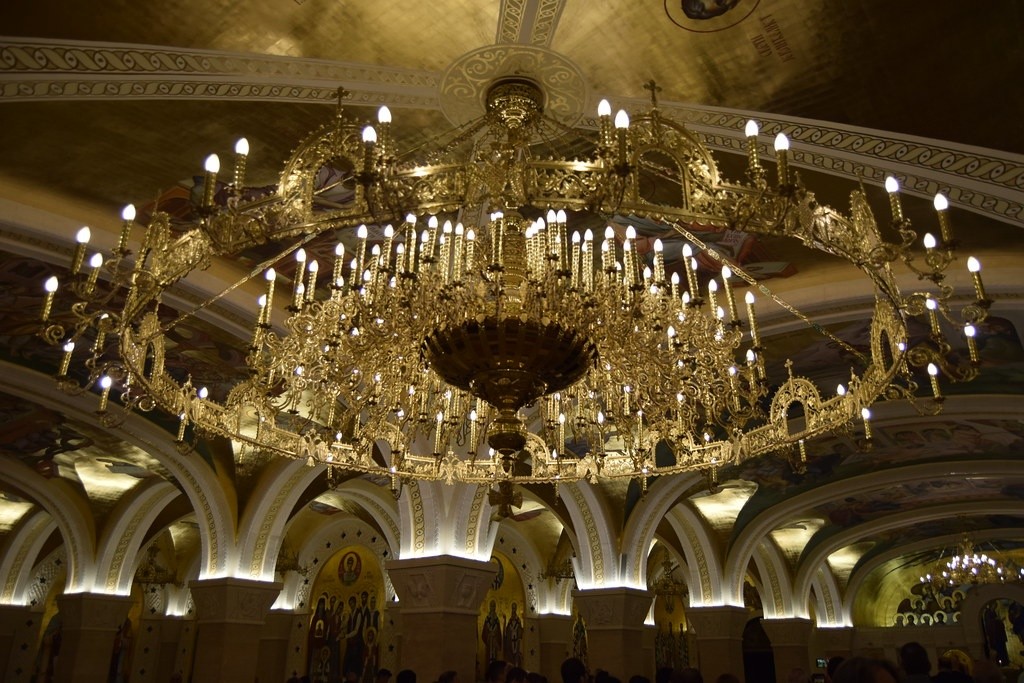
[
  {"x1": 345, "y1": 642, "x2": 1024, "y2": 683},
  {"x1": 954, "y1": 592, "x2": 963, "y2": 601},
  {"x1": 936, "y1": 613, "x2": 945, "y2": 624},
  {"x1": 894, "y1": 616, "x2": 903, "y2": 627},
  {"x1": 1008, "y1": 599, "x2": 1024, "y2": 647},
  {"x1": 169, "y1": 672, "x2": 182, "y2": 683},
  {"x1": 943, "y1": 599, "x2": 952, "y2": 610},
  {"x1": 915, "y1": 600, "x2": 923, "y2": 611},
  {"x1": 984, "y1": 603, "x2": 1011, "y2": 666},
  {"x1": 906, "y1": 615, "x2": 916, "y2": 627},
  {"x1": 923, "y1": 616, "x2": 930, "y2": 626}
]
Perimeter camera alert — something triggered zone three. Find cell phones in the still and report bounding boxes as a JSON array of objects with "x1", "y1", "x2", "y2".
[{"x1": 815, "y1": 658, "x2": 828, "y2": 668}]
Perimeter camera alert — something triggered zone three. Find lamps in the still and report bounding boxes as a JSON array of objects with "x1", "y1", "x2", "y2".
[
  {"x1": 920, "y1": 514, "x2": 1024, "y2": 590},
  {"x1": 32, "y1": 76, "x2": 996, "y2": 494}
]
